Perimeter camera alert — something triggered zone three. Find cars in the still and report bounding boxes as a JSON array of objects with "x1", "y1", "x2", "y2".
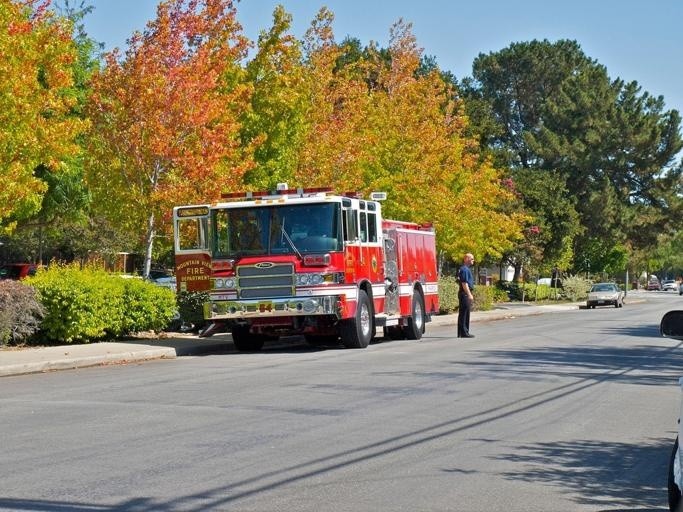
[
  {"x1": 647, "y1": 275, "x2": 677, "y2": 291},
  {"x1": 586, "y1": 283, "x2": 623, "y2": 309}
]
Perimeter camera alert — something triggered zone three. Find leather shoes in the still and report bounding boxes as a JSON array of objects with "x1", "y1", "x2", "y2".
[{"x1": 458, "y1": 334, "x2": 475, "y2": 338}]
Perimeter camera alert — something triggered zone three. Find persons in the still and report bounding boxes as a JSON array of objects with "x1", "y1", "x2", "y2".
[{"x1": 456, "y1": 252, "x2": 476, "y2": 338}]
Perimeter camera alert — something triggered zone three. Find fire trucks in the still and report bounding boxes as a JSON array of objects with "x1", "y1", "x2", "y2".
[{"x1": 172, "y1": 182, "x2": 440, "y2": 350}]
